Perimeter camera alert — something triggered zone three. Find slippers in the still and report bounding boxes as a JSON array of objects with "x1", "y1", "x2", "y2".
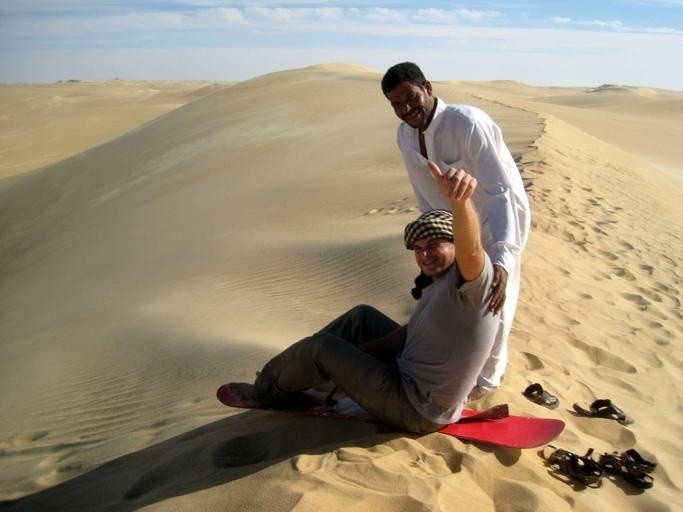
[
  {"x1": 522, "y1": 383, "x2": 559, "y2": 410},
  {"x1": 574, "y1": 399, "x2": 633, "y2": 425}
]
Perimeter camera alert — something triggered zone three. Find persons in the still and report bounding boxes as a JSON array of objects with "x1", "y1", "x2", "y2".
[
  {"x1": 223, "y1": 158, "x2": 501, "y2": 437},
  {"x1": 379, "y1": 60, "x2": 532, "y2": 400}
]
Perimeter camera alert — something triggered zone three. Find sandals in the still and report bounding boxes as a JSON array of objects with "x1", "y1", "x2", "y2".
[
  {"x1": 543, "y1": 445, "x2": 604, "y2": 488},
  {"x1": 591, "y1": 448, "x2": 658, "y2": 489}
]
